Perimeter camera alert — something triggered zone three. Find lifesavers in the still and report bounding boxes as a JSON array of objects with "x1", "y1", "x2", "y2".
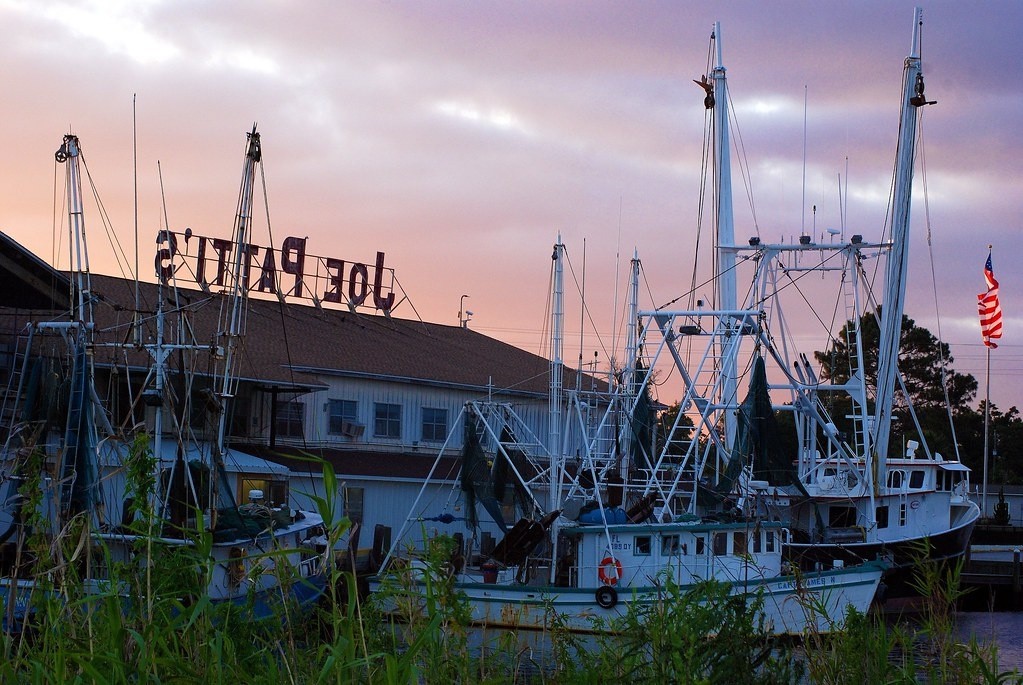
[
  {"x1": 598, "y1": 557, "x2": 622, "y2": 584},
  {"x1": 594, "y1": 585, "x2": 618, "y2": 609}
]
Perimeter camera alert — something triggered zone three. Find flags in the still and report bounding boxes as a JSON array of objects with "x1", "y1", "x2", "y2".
[{"x1": 978, "y1": 249, "x2": 1002, "y2": 350}]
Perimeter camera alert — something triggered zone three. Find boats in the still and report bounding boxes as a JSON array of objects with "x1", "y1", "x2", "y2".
[
  {"x1": 0, "y1": 90, "x2": 330, "y2": 631},
  {"x1": 363, "y1": 243, "x2": 884, "y2": 639},
  {"x1": 570, "y1": 6, "x2": 981, "y2": 617}
]
